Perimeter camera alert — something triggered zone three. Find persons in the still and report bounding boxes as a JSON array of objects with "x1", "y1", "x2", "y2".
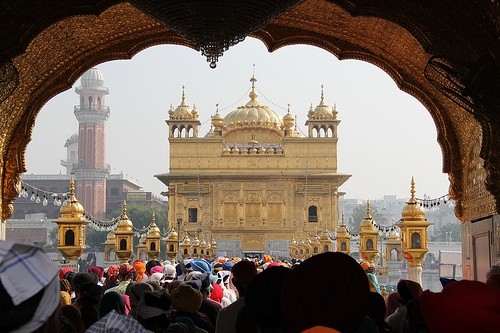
[
  {"x1": 281, "y1": 252, "x2": 369, "y2": 333},
  {"x1": 235, "y1": 266, "x2": 293, "y2": 333},
  {"x1": 215, "y1": 261, "x2": 260, "y2": 333},
  {"x1": 0, "y1": 242, "x2": 500, "y2": 333}
]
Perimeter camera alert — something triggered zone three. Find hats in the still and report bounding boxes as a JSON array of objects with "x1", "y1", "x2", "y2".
[
  {"x1": 88, "y1": 265, "x2": 104, "y2": 280},
  {"x1": 233, "y1": 260, "x2": 257, "y2": 282},
  {"x1": 263, "y1": 254, "x2": 272, "y2": 263},
  {"x1": 150, "y1": 265, "x2": 163, "y2": 274},
  {"x1": 129, "y1": 282, "x2": 154, "y2": 306},
  {"x1": 146, "y1": 259, "x2": 161, "y2": 273},
  {"x1": 170, "y1": 284, "x2": 203, "y2": 312},
  {"x1": 119, "y1": 263, "x2": 135, "y2": 276},
  {"x1": 163, "y1": 263, "x2": 176, "y2": 277},
  {"x1": 60, "y1": 267, "x2": 73, "y2": 278},
  {"x1": 72, "y1": 271, "x2": 99, "y2": 291},
  {"x1": 190, "y1": 259, "x2": 212, "y2": 274},
  {"x1": 210, "y1": 282, "x2": 223, "y2": 301},
  {"x1": 106, "y1": 265, "x2": 119, "y2": 280},
  {"x1": 418, "y1": 280, "x2": 500, "y2": 332},
  {"x1": 222, "y1": 262, "x2": 233, "y2": 270},
  {"x1": 134, "y1": 261, "x2": 146, "y2": 274},
  {"x1": 267, "y1": 262, "x2": 280, "y2": 268}
]
